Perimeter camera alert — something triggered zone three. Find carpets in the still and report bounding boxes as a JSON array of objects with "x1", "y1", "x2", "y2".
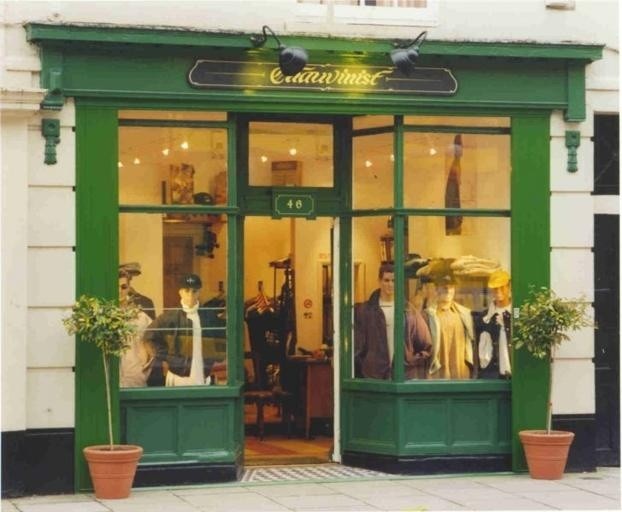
[{"x1": 245, "y1": 457, "x2": 327, "y2": 466}]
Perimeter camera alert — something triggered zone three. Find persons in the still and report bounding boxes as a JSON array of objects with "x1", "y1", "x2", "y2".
[
  {"x1": 420, "y1": 276, "x2": 474, "y2": 381},
  {"x1": 469, "y1": 270, "x2": 511, "y2": 380},
  {"x1": 118, "y1": 272, "x2": 155, "y2": 388},
  {"x1": 354, "y1": 262, "x2": 434, "y2": 380},
  {"x1": 144, "y1": 275, "x2": 226, "y2": 387}
]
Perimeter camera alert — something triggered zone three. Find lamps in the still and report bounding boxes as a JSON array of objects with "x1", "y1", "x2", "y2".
[
  {"x1": 250, "y1": 26, "x2": 307, "y2": 76},
  {"x1": 391, "y1": 31, "x2": 428, "y2": 77}
]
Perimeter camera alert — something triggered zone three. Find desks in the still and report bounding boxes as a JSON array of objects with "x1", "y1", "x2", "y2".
[{"x1": 288, "y1": 359, "x2": 332, "y2": 440}]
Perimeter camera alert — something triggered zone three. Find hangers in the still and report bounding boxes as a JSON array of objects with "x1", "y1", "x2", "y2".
[{"x1": 254, "y1": 291, "x2": 273, "y2": 314}]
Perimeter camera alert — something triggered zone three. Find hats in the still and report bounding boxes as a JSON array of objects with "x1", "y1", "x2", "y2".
[
  {"x1": 435, "y1": 270, "x2": 457, "y2": 286},
  {"x1": 488, "y1": 268, "x2": 510, "y2": 289},
  {"x1": 179, "y1": 273, "x2": 201, "y2": 289}
]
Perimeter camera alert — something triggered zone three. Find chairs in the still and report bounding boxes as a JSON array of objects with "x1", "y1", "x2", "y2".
[{"x1": 245, "y1": 352, "x2": 292, "y2": 440}]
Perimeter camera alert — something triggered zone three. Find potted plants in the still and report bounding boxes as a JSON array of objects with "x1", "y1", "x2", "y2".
[
  {"x1": 511, "y1": 285, "x2": 599, "y2": 480},
  {"x1": 63, "y1": 295, "x2": 143, "y2": 499}
]
